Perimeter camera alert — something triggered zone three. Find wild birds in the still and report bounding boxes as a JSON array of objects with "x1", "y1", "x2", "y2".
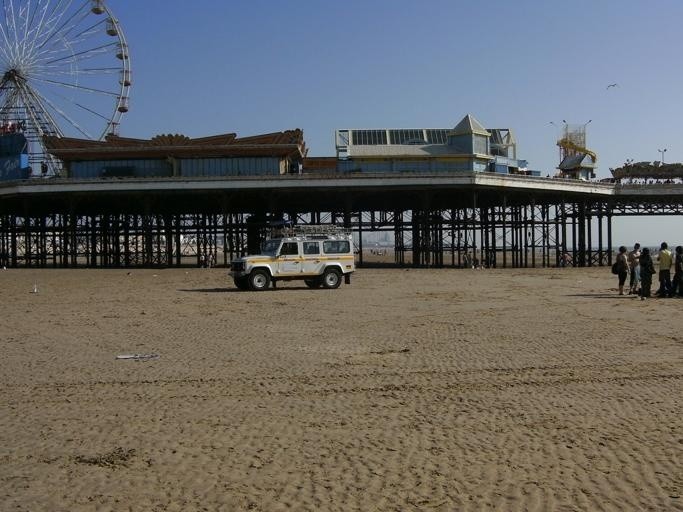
[{"x1": 607, "y1": 84, "x2": 618, "y2": 90}]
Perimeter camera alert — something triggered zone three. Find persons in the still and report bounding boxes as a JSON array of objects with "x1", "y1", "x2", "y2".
[
  {"x1": 616, "y1": 246, "x2": 630, "y2": 296},
  {"x1": 657, "y1": 242, "x2": 672, "y2": 297},
  {"x1": 463, "y1": 251, "x2": 495, "y2": 268},
  {"x1": 668, "y1": 246, "x2": 683, "y2": 297},
  {"x1": 639, "y1": 248, "x2": 653, "y2": 297},
  {"x1": 198, "y1": 252, "x2": 212, "y2": 268},
  {"x1": 41, "y1": 161, "x2": 48, "y2": 178},
  {"x1": 627, "y1": 243, "x2": 641, "y2": 295}
]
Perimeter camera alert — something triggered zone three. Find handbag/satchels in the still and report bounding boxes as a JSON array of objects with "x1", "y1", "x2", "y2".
[{"x1": 612, "y1": 263, "x2": 618, "y2": 274}]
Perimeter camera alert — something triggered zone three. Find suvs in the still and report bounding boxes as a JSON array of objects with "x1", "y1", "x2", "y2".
[{"x1": 228, "y1": 234, "x2": 355, "y2": 290}]
